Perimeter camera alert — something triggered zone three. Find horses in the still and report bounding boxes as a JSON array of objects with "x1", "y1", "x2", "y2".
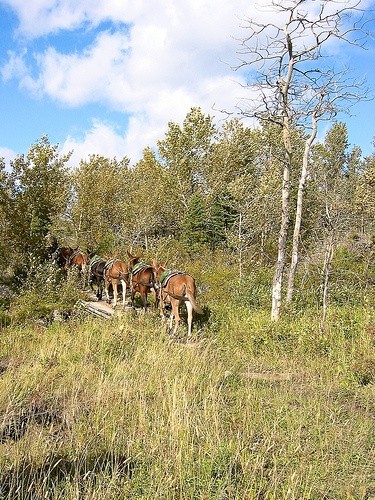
[
  {"x1": 85, "y1": 248, "x2": 108, "y2": 300},
  {"x1": 103, "y1": 255, "x2": 129, "y2": 310},
  {"x1": 154, "y1": 262, "x2": 205, "y2": 336},
  {"x1": 56, "y1": 246, "x2": 70, "y2": 269},
  {"x1": 69, "y1": 246, "x2": 89, "y2": 290},
  {"x1": 126, "y1": 250, "x2": 160, "y2": 312}
]
